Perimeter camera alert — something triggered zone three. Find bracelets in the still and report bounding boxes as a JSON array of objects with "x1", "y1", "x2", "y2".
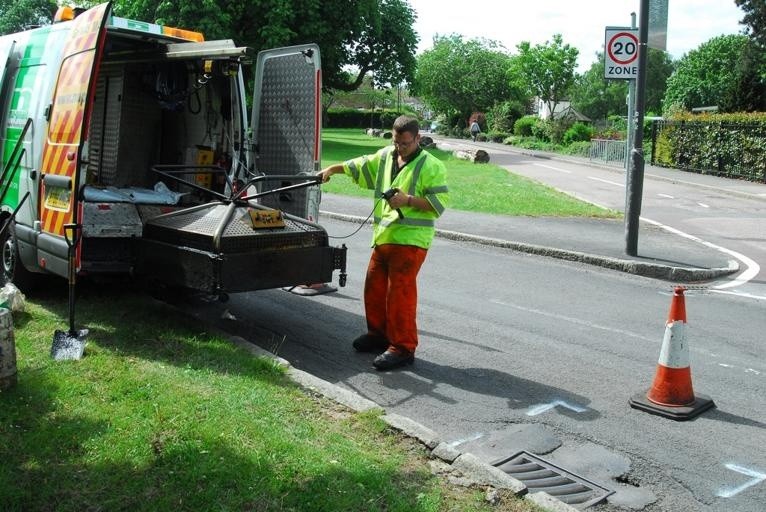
[{"x1": 407, "y1": 196, "x2": 411, "y2": 206}]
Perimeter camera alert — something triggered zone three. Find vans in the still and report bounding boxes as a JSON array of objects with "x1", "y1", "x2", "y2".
[{"x1": 0, "y1": 1, "x2": 348, "y2": 306}]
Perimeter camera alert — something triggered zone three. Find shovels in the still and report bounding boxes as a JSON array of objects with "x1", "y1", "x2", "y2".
[{"x1": 49, "y1": 224, "x2": 90, "y2": 362}]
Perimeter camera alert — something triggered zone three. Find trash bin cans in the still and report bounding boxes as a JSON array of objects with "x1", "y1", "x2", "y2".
[{"x1": 1, "y1": 307, "x2": 21, "y2": 394}]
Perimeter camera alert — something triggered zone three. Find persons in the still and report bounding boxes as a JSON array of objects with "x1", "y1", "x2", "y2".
[
  {"x1": 316, "y1": 115, "x2": 449, "y2": 371},
  {"x1": 470, "y1": 119, "x2": 481, "y2": 142}
]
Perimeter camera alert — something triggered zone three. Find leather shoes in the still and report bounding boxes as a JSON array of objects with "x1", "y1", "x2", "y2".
[
  {"x1": 373, "y1": 350, "x2": 414, "y2": 371},
  {"x1": 352, "y1": 335, "x2": 388, "y2": 353}
]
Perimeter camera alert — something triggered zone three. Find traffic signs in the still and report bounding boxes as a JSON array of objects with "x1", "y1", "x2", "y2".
[{"x1": 604, "y1": 26, "x2": 640, "y2": 80}]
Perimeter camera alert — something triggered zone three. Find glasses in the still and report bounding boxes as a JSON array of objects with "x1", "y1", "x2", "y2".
[{"x1": 391, "y1": 137, "x2": 415, "y2": 149}]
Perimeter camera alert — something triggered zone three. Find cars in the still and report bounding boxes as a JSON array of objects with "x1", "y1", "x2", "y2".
[{"x1": 428, "y1": 121, "x2": 442, "y2": 133}]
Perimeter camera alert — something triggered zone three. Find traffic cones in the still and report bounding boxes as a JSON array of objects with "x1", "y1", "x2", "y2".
[
  {"x1": 278, "y1": 281, "x2": 339, "y2": 298},
  {"x1": 629, "y1": 286, "x2": 717, "y2": 421}
]
